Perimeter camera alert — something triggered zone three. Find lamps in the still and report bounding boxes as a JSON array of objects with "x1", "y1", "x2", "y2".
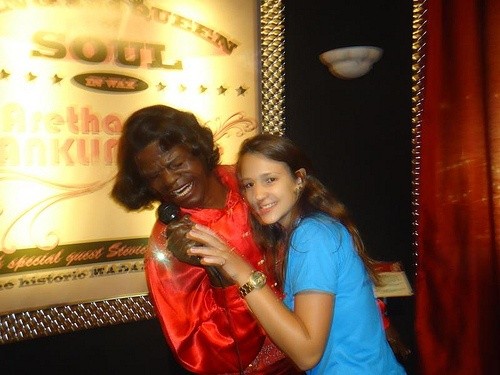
[{"x1": 320, "y1": 46, "x2": 383, "y2": 80}]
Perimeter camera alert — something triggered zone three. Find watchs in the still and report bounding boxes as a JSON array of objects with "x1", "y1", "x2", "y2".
[{"x1": 239, "y1": 271, "x2": 267, "y2": 297}]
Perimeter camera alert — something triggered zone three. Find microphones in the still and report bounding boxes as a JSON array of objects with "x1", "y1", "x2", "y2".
[{"x1": 158, "y1": 201, "x2": 223, "y2": 287}]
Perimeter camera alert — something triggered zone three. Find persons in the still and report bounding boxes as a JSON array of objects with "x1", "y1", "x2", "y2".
[
  {"x1": 187, "y1": 134, "x2": 407, "y2": 375},
  {"x1": 109, "y1": 105, "x2": 389, "y2": 375}
]
